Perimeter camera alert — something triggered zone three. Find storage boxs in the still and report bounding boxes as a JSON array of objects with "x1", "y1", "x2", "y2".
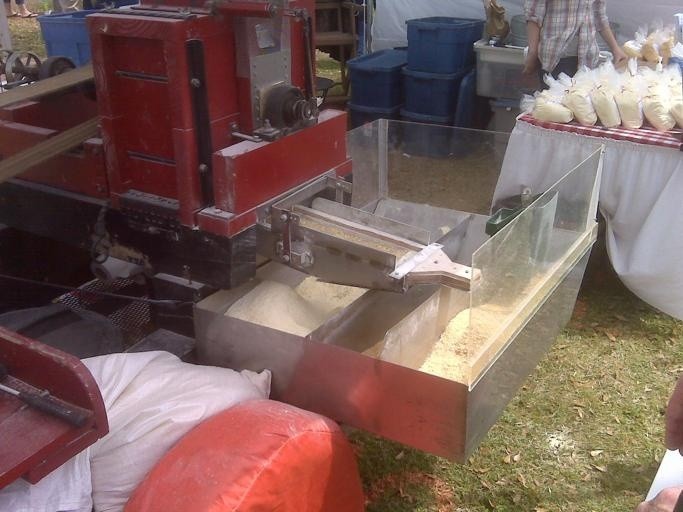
[{"x1": 345, "y1": 14, "x2": 541, "y2": 163}]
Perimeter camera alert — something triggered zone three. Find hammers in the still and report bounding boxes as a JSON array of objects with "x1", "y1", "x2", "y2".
[{"x1": 0, "y1": 363, "x2": 87, "y2": 429}]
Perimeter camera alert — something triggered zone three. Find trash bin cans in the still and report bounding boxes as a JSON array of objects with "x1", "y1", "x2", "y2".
[{"x1": 38, "y1": 11, "x2": 97, "y2": 69}]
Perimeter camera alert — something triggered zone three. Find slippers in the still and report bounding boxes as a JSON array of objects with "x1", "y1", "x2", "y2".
[{"x1": 7, "y1": 12, "x2": 38, "y2": 18}]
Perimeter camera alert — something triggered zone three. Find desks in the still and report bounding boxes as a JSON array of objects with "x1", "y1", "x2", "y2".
[{"x1": 515, "y1": 112, "x2": 682, "y2": 287}]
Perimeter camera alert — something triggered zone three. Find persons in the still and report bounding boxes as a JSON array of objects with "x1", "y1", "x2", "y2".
[
  {"x1": 522, "y1": 0, "x2": 628, "y2": 93},
  {"x1": 635, "y1": 371, "x2": 683, "y2": 512},
  {"x1": 3, "y1": 0, "x2": 39, "y2": 18}
]
